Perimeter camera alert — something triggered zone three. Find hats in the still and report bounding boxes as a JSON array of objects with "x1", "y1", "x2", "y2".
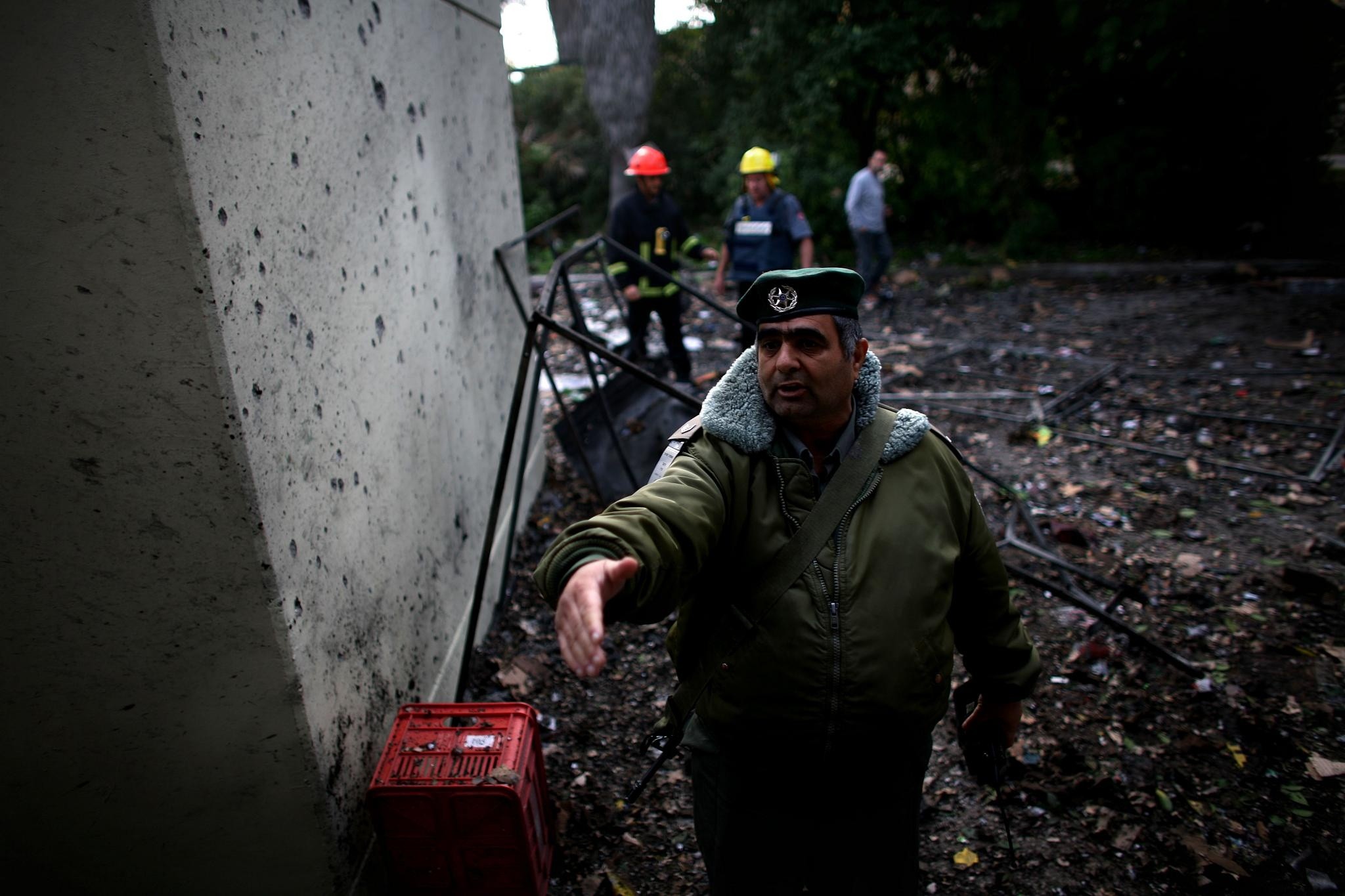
[{"x1": 735, "y1": 267, "x2": 867, "y2": 327}]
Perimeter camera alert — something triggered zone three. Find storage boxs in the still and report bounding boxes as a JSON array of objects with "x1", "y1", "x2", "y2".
[{"x1": 365, "y1": 702, "x2": 555, "y2": 896}]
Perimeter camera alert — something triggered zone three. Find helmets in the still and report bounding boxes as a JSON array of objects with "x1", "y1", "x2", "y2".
[
  {"x1": 740, "y1": 146, "x2": 775, "y2": 175},
  {"x1": 623, "y1": 146, "x2": 669, "y2": 176}
]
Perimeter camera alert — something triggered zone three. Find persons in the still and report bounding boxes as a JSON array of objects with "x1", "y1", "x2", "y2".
[
  {"x1": 715, "y1": 147, "x2": 813, "y2": 351},
  {"x1": 604, "y1": 146, "x2": 720, "y2": 382},
  {"x1": 843, "y1": 149, "x2": 893, "y2": 296},
  {"x1": 532, "y1": 267, "x2": 1042, "y2": 896}
]
